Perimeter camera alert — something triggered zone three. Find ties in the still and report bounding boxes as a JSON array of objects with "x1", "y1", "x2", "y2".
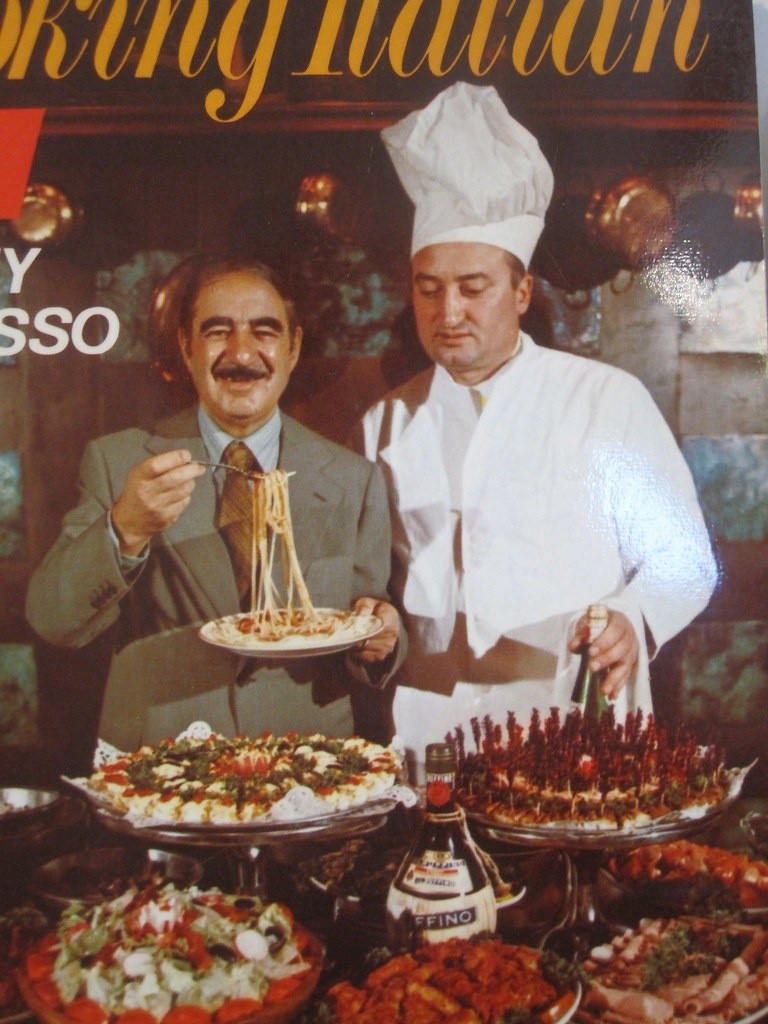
[{"x1": 219, "y1": 442, "x2": 259, "y2": 613}]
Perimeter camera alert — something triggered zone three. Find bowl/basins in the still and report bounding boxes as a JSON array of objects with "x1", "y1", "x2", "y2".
[{"x1": 29, "y1": 847, "x2": 203, "y2": 906}]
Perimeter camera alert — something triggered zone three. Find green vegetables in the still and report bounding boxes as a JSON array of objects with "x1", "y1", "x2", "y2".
[{"x1": 503, "y1": 907, "x2": 745, "y2": 1024}]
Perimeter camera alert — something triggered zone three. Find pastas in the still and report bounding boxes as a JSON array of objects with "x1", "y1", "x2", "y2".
[{"x1": 245, "y1": 468, "x2": 349, "y2": 641}]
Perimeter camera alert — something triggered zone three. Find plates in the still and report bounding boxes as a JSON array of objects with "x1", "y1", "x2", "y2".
[
  {"x1": 198, "y1": 608, "x2": 384, "y2": 659},
  {"x1": 15, "y1": 896, "x2": 324, "y2": 1023},
  {"x1": 511, "y1": 948, "x2": 582, "y2": 1023}
]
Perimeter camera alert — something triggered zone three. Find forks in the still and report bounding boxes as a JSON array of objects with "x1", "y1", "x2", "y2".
[{"x1": 185, "y1": 458, "x2": 297, "y2": 479}]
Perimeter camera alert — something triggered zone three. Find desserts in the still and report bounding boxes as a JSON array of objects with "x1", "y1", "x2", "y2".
[{"x1": 444, "y1": 706, "x2": 733, "y2": 831}]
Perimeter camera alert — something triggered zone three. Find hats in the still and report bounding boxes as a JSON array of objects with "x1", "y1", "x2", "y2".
[{"x1": 380, "y1": 81, "x2": 554, "y2": 275}]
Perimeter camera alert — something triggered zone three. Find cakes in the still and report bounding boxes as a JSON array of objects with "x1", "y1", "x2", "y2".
[{"x1": 88, "y1": 726, "x2": 400, "y2": 824}]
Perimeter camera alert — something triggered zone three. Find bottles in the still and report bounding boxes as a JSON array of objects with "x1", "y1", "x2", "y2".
[
  {"x1": 385, "y1": 743, "x2": 497, "y2": 943},
  {"x1": 570, "y1": 606, "x2": 619, "y2": 726}
]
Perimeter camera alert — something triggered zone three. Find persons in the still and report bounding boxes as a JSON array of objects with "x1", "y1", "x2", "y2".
[
  {"x1": 24, "y1": 254, "x2": 408, "y2": 752},
  {"x1": 351, "y1": 205, "x2": 715, "y2": 771}
]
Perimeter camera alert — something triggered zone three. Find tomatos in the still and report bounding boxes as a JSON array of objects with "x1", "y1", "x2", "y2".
[{"x1": 536, "y1": 990, "x2": 574, "y2": 1024}]
